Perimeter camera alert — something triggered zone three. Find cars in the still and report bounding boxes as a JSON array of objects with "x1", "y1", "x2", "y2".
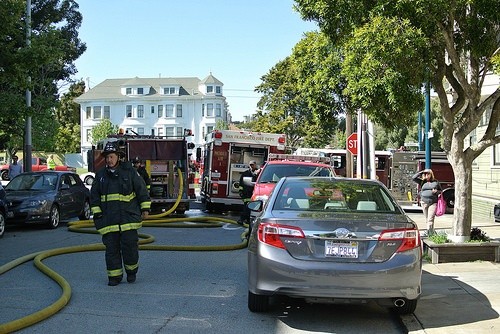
[
  {"x1": 79, "y1": 171, "x2": 96, "y2": 185},
  {"x1": 245, "y1": 153, "x2": 423, "y2": 316},
  {"x1": 0, "y1": 171, "x2": 92, "y2": 238}
]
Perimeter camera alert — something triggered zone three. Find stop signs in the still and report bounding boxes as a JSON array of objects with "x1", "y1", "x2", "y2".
[{"x1": 346, "y1": 133, "x2": 358, "y2": 155}]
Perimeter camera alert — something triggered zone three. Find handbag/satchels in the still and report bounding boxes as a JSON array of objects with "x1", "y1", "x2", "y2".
[{"x1": 437, "y1": 192, "x2": 446, "y2": 216}]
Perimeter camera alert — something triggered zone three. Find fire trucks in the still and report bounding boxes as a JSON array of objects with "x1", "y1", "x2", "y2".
[
  {"x1": 293, "y1": 146, "x2": 455, "y2": 216},
  {"x1": 86, "y1": 129, "x2": 195, "y2": 216},
  {"x1": 197, "y1": 129, "x2": 287, "y2": 214}
]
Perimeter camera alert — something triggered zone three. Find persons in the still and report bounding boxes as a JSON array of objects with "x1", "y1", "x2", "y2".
[
  {"x1": 47, "y1": 154, "x2": 59, "y2": 171},
  {"x1": 132, "y1": 156, "x2": 150, "y2": 196},
  {"x1": 8, "y1": 156, "x2": 22, "y2": 182},
  {"x1": 412, "y1": 169, "x2": 443, "y2": 237},
  {"x1": 235, "y1": 160, "x2": 266, "y2": 228},
  {"x1": 89, "y1": 143, "x2": 152, "y2": 286}
]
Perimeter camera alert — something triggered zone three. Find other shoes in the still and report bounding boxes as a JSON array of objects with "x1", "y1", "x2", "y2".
[
  {"x1": 108, "y1": 279, "x2": 120, "y2": 286},
  {"x1": 127, "y1": 273, "x2": 136, "y2": 282},
  {"x1": 237, "y1": 220, "x2": 244, "y2": 226}
]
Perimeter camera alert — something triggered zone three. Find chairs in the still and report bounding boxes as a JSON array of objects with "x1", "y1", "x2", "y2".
[
  {"x1": 287, "y1": 188, "x2": 307, "y2": 208},
  {"x1": 30, "y1": 176, "x2": 45, "y2": 187},
  {"x1": 48, "y1": 178, "x2": 62, "y2": 190},
  {"x1": 348, "y1": 191, "x2": 372, "y2": 208}
]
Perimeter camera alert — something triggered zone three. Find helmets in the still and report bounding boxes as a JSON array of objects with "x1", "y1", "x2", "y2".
[
  {"x1": 132, "y1": 157, "x2": 142, "y2": 163},
  {"x1": 250, "y1": 160, "x2": 257, "y2": 164},
  {"x1": 100, "y1": 142, "x2": 126, "y2": 159}
]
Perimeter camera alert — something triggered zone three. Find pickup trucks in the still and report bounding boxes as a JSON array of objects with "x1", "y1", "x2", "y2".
[{"x1": 0, "y1": 157, "x2": 76, "y2": 181}]
